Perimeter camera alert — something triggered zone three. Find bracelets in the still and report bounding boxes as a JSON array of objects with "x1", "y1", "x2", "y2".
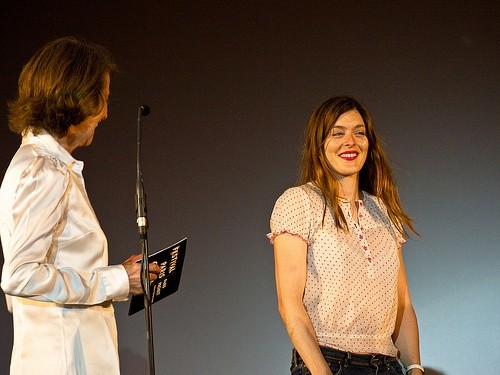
[{"x1": 404, "y1": 364, "x2": 426, "y2": 373}]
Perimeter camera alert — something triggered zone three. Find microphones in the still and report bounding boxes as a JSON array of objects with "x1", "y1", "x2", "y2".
[{"x1": 136, "y1": 104, "x2": 151, "y2": 237}]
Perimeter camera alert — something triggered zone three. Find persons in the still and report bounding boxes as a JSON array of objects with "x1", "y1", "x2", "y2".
[
  {"x1": 265, "y1": 96, "x2": 426, "y2": 375},
  {"x1": 0, "y1": 38, "x2": 161, "y2": 375}
]
